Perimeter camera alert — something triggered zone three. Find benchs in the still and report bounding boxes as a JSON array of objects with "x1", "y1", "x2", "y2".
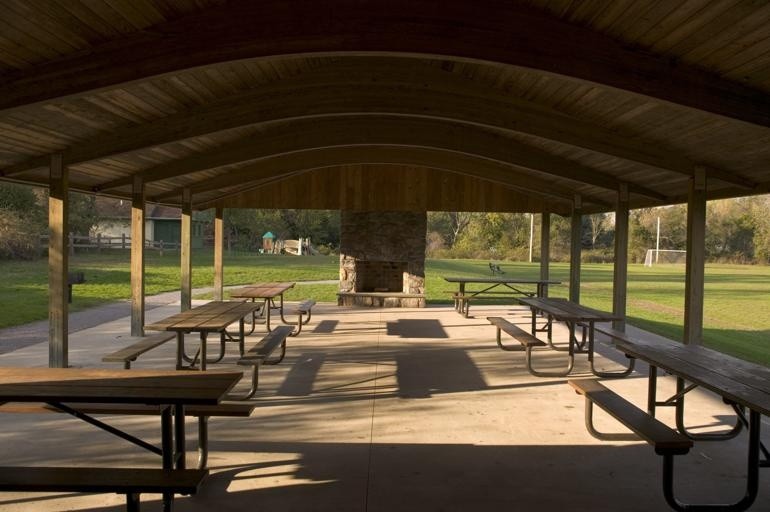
[
  {"x1": 0, "y1": 401, "x2": 257, "y2": 469},
  {"x1": 235, "y1": 326, "x2": 295, "y2": 401},
  {"x1": 296, "y1": 299, "x2": 314, "y2": 335},
  {"x1": 101, "y1": 329, "x2": 180, "y2": 369},
  {"x1": 0, "y1": 457, "x2": 209, "y2": 511},
  {"x1": 567, "y1": 378, "x2": 695, "y2": 512},
  {"x1": 487, "y1": 316, "x2": 546, "y2": 380},
  {"x1": 580, "y1": 321, "x2": 639, "y2": 376}
]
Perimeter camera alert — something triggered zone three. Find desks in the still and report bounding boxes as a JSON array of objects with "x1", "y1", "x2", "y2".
[
  {"x1": 229, "y1": 282, "x2": 296, "y2": 333},
  {"x1": 1, "y1": 369, "x2": 244, "y2": 467},
  {"x1": 141, "y1": 301, "x2": 265, "y2": 370},
  {"x1": 444, "y1": 277, "x2": 561, "y2": 315},
  {"x1": 518, "y1": 297, "x2": 624, "y2": 377},
  {"x1": 616, "y1": 342, "x2": 769, "y2": 511}
]
